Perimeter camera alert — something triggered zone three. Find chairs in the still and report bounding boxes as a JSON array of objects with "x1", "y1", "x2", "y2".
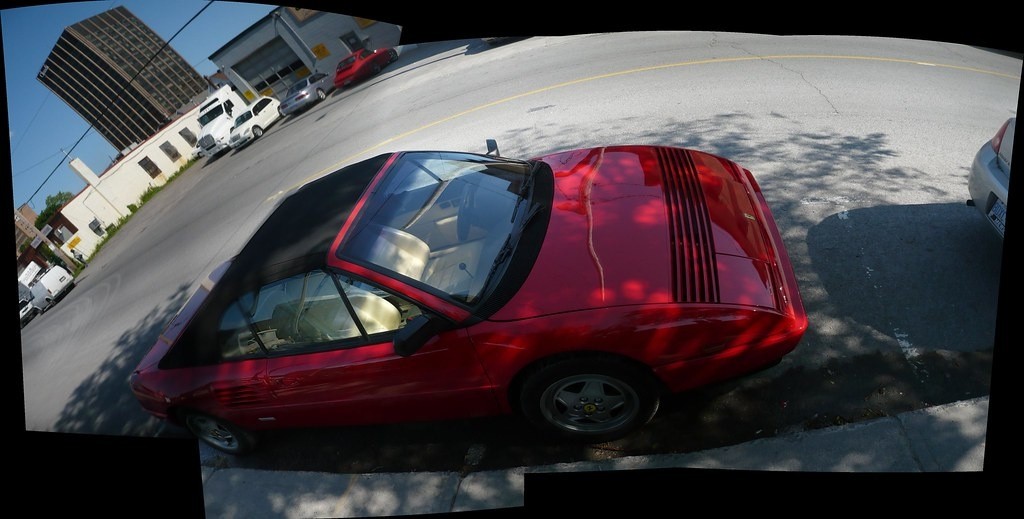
[
  {"x1": 271, "y1": 275, "x2": 400, "y2": 341},
  {"x1": 368, "y1": 225, "x2": 486, "y2": 295}
]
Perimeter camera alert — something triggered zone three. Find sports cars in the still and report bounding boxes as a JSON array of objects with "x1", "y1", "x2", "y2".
[
  {"x1": 332, "y1": 46, "x2": 400, "y2": 90},
  {"x1": 128, "y1": 138, "x2": 810, "y2": 456}
]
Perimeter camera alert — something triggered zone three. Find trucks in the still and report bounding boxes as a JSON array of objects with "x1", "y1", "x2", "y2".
[{"x1": 192, "y1": 84, "x2": 255, "y2": 159}]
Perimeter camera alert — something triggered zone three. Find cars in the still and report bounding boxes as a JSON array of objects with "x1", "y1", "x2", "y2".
[
  {"x1": 965, "y1": 111, "x2": 1017, "y2": 246},
  {"x1": 18, "y1": 298, "x2": 35, "y2": 329},
  {"x1": 227, "y1": 93, "x2": 282, "y2": 151},
  {"x1": 278, "y1": 70, "x2": 333, "y2": 118}
]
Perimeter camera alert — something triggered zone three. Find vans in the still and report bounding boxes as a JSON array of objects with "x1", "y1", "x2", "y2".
[{"x1": 27, "y1": 265, "x2": 76, "y2": 315}]
